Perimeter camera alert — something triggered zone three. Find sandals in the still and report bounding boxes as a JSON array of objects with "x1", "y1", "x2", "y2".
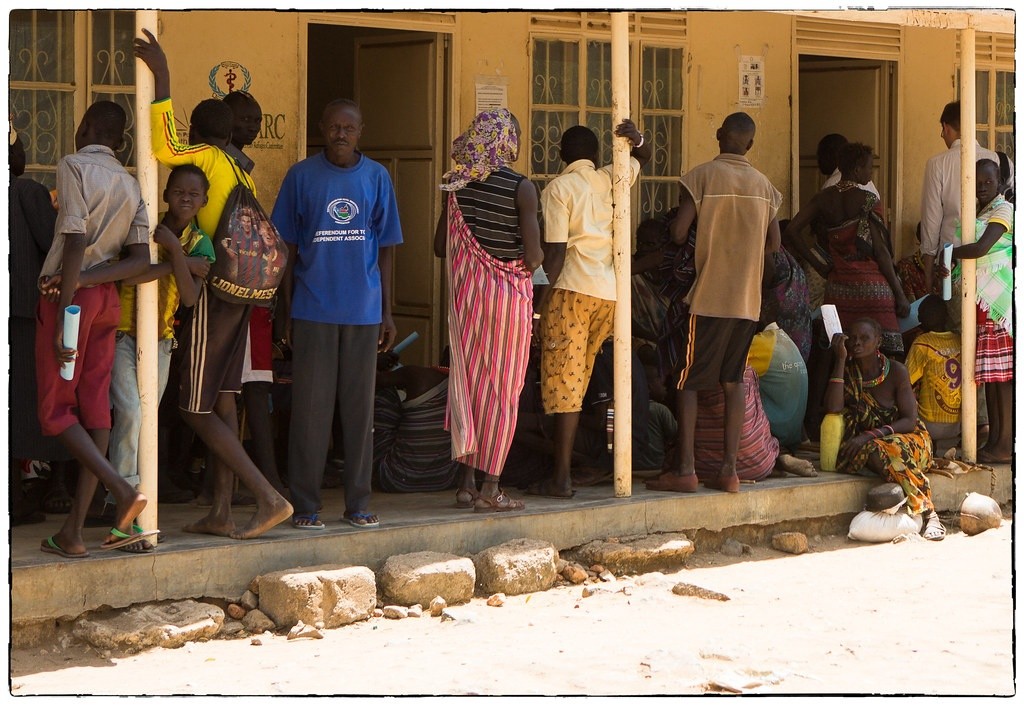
[
  {"x1": 474, "y1": 488, "x2": 525, "y2": 513},
  {"x1": 119, "y1": 540, "x2": 154, "y2": 553},
  {"x1": 454, "y1": 487, "x2": 480, "y2": 508}
]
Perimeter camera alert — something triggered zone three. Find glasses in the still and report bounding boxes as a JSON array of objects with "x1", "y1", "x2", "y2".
[{"x1": 941, "y1": 122, "x2": 949, "y2": 137}]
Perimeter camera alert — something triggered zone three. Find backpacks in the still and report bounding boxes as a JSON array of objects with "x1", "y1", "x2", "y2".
[{"x1": 194, "y1": 150, "x2": 289, "y2": 307}]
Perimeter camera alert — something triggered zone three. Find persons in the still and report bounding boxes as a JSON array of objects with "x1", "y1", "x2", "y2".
[
  {"x1": 523, "y1": 118, "x2": 651, "y2": 502},
  {"x1": 8, "y1": 27, "x2": 294, "y2": 559},
  {"x1": 270, "y1": 97, "x2": 404, "y2": 529},
  {"x1": 435, "y1": 108, "x2": 545, "y2": 513},
  {"x1": 572, "y1": 101, "x2": 1015, "y2": 541}
]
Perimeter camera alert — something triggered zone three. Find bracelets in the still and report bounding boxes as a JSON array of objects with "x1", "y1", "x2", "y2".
[
  {"x1": 864, "y1": 430, "x2": 878, "y2": 439},
  {"x1": 629, "y1": 131, "x2": 643, "y2": 148},
  {"x1": 883, "y1": 425, "x2": 895, "y2": 434},
  {"x1": 874, "y1": 427, "x2": 885, "y2": 437},
  {"x1": 830, "y1": 378, "x2": 845, "y2": 383}
]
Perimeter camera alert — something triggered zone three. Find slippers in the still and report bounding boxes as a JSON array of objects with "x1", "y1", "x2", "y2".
[
  {"x1": 100, "y1": 525, "x2": 162, "y2": 550},
  {"x1": 922, "y1": 521, "x2": 945, "y2": 541},
  {"x1": 342, "y1": 512, "x2": 380, "y2": 528},
  {"x1": 976, "y1": 450, "x2": 1012, "y2": 463},
  {"x1": 526, "y1": 479, "x2": 573, "y2": 499},
  {"x1": 293, "y1": 514, "x2": 325, "y2": 530},
  {"x1": 573, "y1": 472, "x2": 614, "y2": 486},
  {"x1": 40, "y1": 536, "x2": 89, "y2": 558}
]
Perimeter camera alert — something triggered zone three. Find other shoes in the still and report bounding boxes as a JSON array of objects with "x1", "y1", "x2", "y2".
[
  {"x1": 41, "y1": 487, "x2": 74, "y2": 512},
  {"x1": 646, "y1": 472, "x2": 699, "y2": 493},
  {"x1": 704, "y1": 471, "x2": 740, "y2": 493},
  {"x1": 158, "y1": 490, "x2": 193, "y2": 503},
  {"x1": 86, "y1": 502, "x2": 119, "y2": 526}
]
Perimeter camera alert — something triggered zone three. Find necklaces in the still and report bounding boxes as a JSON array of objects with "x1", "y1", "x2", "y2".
[
  {"x1": 836, "y1": 179, "x2": 859, "y2": 192},
  {"x1": 849, "y1": 351, "x2": 891, "y2": 387}
]
